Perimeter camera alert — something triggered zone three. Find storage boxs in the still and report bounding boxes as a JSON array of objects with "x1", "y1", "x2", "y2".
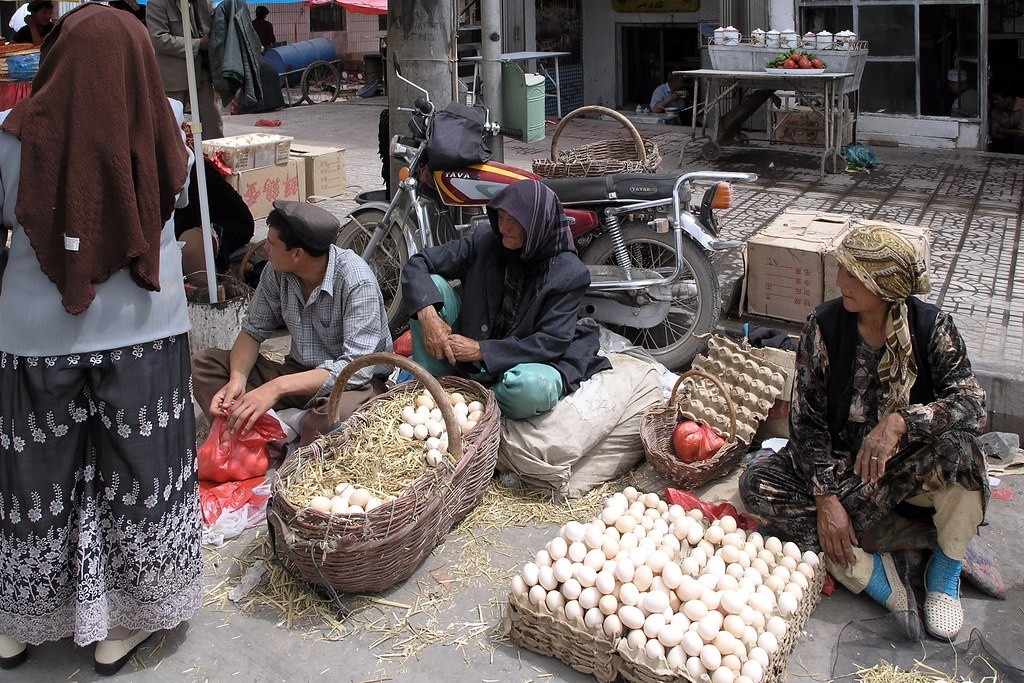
[
  {"x1": 775, "y1": 106, "x2": 853, "y2": 145},
  {"x1": 747, "y1": 209, "x2": 852, "y2": 323},
  {"x1": 202, "y1": 133, "x2": 347, "y2": 220},
  {"x1": 823, "y1": 218, "x2": 929, "y2": 302}
]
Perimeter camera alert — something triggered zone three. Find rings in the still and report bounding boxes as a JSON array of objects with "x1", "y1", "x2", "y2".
[{"x1": 870, "y1": 455, "x2": 878, "y2": 460}]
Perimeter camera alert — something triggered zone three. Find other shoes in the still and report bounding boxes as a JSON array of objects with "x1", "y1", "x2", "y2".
[
  {"x1": 92, "y1": 622, "x2": 155, "y2": 675},
  {"x1": 879, "y1": 550, "x2": 919, "y2": 641},
  {"x1": 0, "y1": 632, "x2": 28, "y2": 670},
  {"x1": 924, "y1": 554, "x2": 964, "y2": 642}
]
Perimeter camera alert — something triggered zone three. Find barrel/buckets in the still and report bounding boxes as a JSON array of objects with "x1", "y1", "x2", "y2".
[{"x1": 263, "y1": 37, "x2": 336, "y2": 88}]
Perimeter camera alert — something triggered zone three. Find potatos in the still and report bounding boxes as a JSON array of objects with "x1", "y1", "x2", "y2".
[{"x1": 783, "y1": 54, "x2": 823, "y2": 70}]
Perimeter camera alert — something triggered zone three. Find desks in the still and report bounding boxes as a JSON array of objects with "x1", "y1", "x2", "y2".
[
  {"x1": 461, "y1": 51, "x2": 571, "y2": 122},
  {"x1": 600, "y1": 109, "x2": 680, "y2": 126},
  {"x1": 672, "y1": 68, "x2": 855, "y2": 184}
]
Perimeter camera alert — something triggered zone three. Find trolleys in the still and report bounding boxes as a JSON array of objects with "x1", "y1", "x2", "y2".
[{"x1": 263, "y1": 38, "x2": 352, "y2": 107}]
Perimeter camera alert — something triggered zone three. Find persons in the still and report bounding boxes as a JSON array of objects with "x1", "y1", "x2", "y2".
[
  {"x1": 191, "y1": 197, "x2": 394, "y2": 459},
  {"x1": 650, "y1": 68, "x2": 702, "y2": 126},
  {"x1": 947, "y1": 68, "x2": 994, "y2": 151},
  {"x1": 109, "y1": 0, "x2": 147, "y2": 28},
  {"x1": 400, "y1": 178, "x2": 612, "y2": 421},
  {"x1": 145, "y1": 0, "x2": 225, "y2": 140},
  {"x1": 0, "y1": 1, "x2": 203, "y2": 677},
  {"x1": 175, "y1": 153, "x2": 255, "y2": 303},
  {"x1": 15, "y1": 0, "x2": 54, "y2": 43},
  {"x1": 252, "y1": 5, "x2": 277, "y2": 48},
  {"x1": 738, "y1": 224, "x2": 991, "y2": 642}
]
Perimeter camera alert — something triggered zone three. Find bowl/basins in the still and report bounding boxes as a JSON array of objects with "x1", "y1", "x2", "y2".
[
  {"x1": 677, "y1": 90, "x2": 689, "y2": 98},
  {"x1": 665, "y1": 107, "x2": 678, "y2": 115},
  {"x1": 356, "y1": 81, "x2": 378, "y2": 98}
]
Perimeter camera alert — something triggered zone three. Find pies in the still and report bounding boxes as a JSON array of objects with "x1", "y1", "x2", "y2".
[{"x1": 0, "y1": 43, "x2": 41, "y2": 79}]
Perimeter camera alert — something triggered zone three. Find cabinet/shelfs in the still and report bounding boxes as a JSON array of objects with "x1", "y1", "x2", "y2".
[{"x1": 702, "y1": 37, "x2": 868, "y2": 174}]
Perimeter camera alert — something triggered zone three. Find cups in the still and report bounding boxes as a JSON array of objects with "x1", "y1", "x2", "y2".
[
  {"x1": 803, "y1": 30, "x2": 856, "y2": 51},
  {"x1": 750, "y1": 28, "x2": 801, "y2": 49},
  {"x1": 714, "y1": 26, "x2": 741, "y2": 45},
  {"x1": 636, "y1": 104, "x2": 642, "y2": 113},
  {"x1": 642, "y1": 108, "x2": 650, "y2": 114}
]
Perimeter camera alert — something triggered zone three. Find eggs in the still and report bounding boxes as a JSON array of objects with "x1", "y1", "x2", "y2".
[
  {"x1": 197, "y1": 428, "x2": 268, "y2": 482},
  {"x1": 204, "y1": 135, "x2": 282, "y2": 146},
  {"x1": 308, "y1": 483, "x2": 382, "y2": 514},
  {"x1": 399, "y1": 388, "x2": 484, "y2": 466},
  {"x1": 511, "y1": 487, "x2": 819, "y2": 683}
]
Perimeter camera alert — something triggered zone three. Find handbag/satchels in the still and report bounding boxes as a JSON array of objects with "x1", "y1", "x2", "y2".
[{"x1": 426, "y1": 102, "x2": 492, "y2": 172}]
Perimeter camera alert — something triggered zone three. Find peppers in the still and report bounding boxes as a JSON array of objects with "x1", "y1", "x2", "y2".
[{"x1": 765, "y1": 48, "x2": 827, "y2": 67}]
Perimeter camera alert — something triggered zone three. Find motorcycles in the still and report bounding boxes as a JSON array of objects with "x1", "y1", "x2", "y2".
[{"x1": 335, "y1": 50, "x2": 758, "y2": 370}]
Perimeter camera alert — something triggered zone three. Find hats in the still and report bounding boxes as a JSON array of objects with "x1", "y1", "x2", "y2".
[
  {"x1": 256, "y1": 6, "x2": 269, "y2": 15},
  {"x1": 947, "y1": 69, "x2": 967, "y2": 81},
  {"x1": 272, "y1": 200, "x2": 340, "y2": 250}
]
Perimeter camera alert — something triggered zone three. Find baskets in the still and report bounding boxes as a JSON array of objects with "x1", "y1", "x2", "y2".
[
  {"x1": 639, "y1": 370, "x2": 750, "y2": 489},
  {"x1": 531, "y1": 104, "x2": 661, "y2": 180},
  {"x1": 267, "y1": 351, "x2": 500, "y2": 591}
]
